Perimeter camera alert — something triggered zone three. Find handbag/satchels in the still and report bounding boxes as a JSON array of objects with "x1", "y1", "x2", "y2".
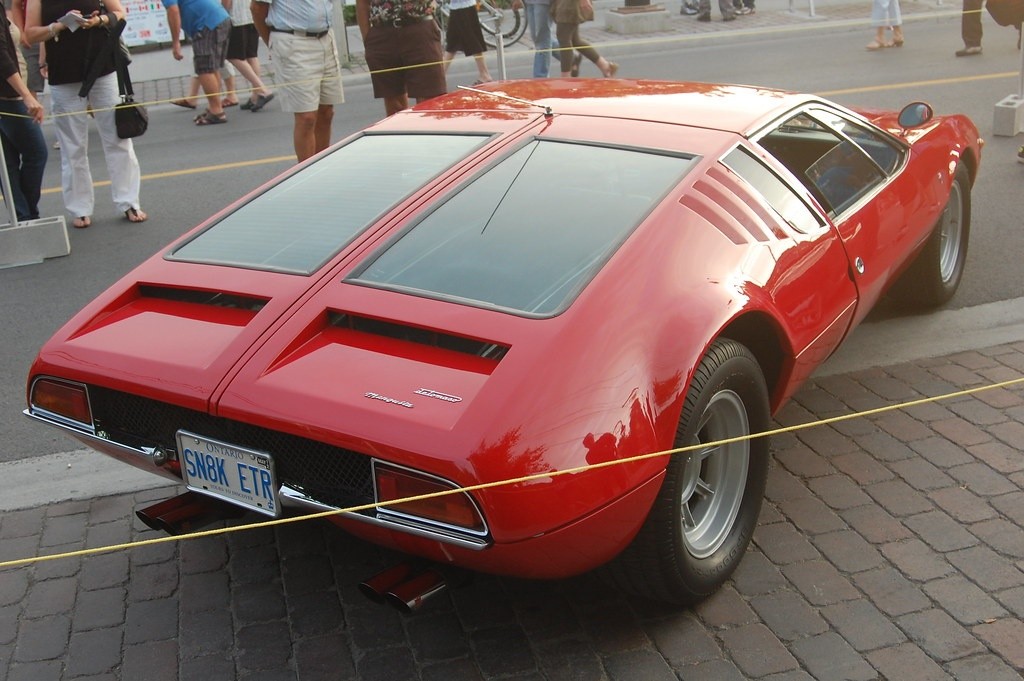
[{"x1": 115, "y1": 99, "x2": 148, "y2": 138}]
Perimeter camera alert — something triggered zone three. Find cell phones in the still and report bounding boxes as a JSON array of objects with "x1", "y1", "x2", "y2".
[{"x1": 82, "y1": 14, "x2": 92, "y2": 19}]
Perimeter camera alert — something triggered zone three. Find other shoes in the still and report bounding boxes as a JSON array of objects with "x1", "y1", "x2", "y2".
[{"x1": 955, "y1": 45, "x2": 984, "y2": 56}]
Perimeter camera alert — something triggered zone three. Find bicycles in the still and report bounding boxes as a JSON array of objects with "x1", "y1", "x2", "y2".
[{"x1": 425, "y1": 0, "x2": 529, "y2": 49}]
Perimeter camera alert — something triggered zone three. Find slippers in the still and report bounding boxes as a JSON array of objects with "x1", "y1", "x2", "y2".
[
  {"x1": 240, "y1": 97, "x2": 254, "y2": 110},
  {"x1": 172, "y1": 99, "x2": 196, "y2": 109},
  {"x1": 472, "y1": 79, "x2": 484, "y2": 86},
  {"x1": 251, "y1": 92, "x2": 274, "y2": 111},
  {"x1": 222, "y1": 97, "x2": 238, "y2": 108}
]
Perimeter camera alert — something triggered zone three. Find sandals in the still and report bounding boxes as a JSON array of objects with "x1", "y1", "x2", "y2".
[
  {"x1": 74, "y1": 216, "x2": 89, "y2": 228},
  {"x1": 193, "y1": 108, "x2": 228, "y2": 125},
  {"x1": 124, "y1": 206, "x2": 141, "y2": 222},
  {"x1": 570, "y1": 52, "x2": 582, "y2": 77},
  {"x1": 604, "y1": 61, "x2": 619, "y2": 78}
]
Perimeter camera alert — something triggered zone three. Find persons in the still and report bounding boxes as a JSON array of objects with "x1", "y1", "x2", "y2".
[
  {"x1": 443, "y1": 0, "x2": 491, "y2": 86},
  {"x1": 512, "y1": 0, "x2": 582, "y2": 78},
  {"x1": 162, "y1": 0, "x2": 231, "y2": 124},
  {"x1": 551, "y1": 0, "x2": 618, "y2": 78},
  {"x1": 11, "y1": 0, "x2": 147, "y2": 229},
  {"x1": 356, "y1": 0, "x2": 447, "y2": 115},
  {"x1": 865, "y1": 0, "x2": 904, "y2": 49},
  {"x1": 250, "y1": 0, "x2": 345, "y2": 164},
  {"x1": 170, "y1": 0, "x2": 275, "y2": 110},
  {"x1": 681, "y1": 0, "x2": 756, "y2": 22},
  {"x1": 0, "y1": 0, "x2": 49, "y2": 225},
  {"x1": 955, "y1": 0, "x2": 1024, "y2": 56}
]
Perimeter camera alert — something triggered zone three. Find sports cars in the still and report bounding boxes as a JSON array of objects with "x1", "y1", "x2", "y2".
[{"x1": 23, "y1": 76, "x2": 985, "y2": 616}]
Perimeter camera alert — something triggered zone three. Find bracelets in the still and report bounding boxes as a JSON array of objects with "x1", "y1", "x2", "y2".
[
  {"x1": 98, "y1": 16, "x2": 104, "y2": 28},
  {"x1": 39, "y1": 63, "x2": 48, "y2": 69},
  {"x1": 49, "y1": 23, "x2": 59, "y2": 41}
]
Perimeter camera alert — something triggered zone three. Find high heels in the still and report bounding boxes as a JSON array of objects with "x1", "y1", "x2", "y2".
[
  {"x1": 867, "y1": 39, "x2": 884, "y2": 49},
  {"x1": 885, "y1": 36, "x2": 905, "y2": 48}
]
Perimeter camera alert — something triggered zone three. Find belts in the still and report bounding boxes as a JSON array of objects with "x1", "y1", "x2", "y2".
[
  {"x1": 374, "y1": 14, "x2": 433, "y2": 29},
  {"x1": 272, "y1": 26, "x2": 329, "y2": 39}
]
